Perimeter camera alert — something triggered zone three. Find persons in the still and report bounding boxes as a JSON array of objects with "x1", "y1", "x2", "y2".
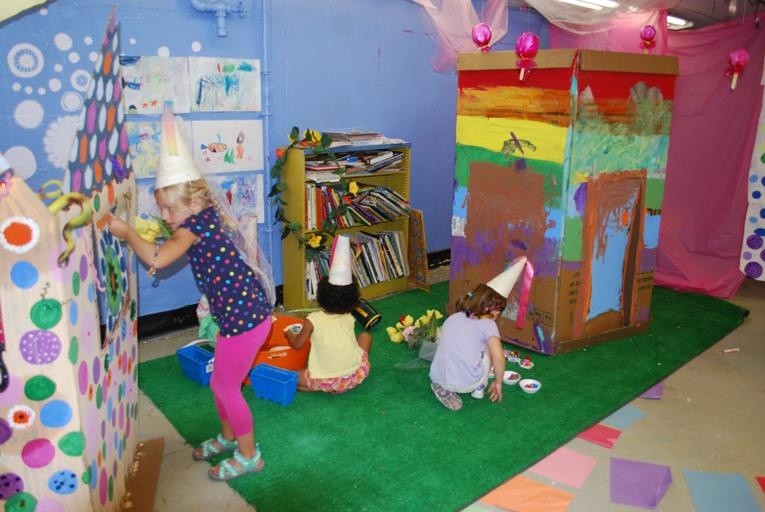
[
  {"x1": 282, "y1": 236, "x2": 372, "y2": 392},
  {"x1": 427, "y1": 256, "x2": 535, "y2": 412},
  {"x1": 107, "y1": 110, "x2": 272, "y2": 481}
]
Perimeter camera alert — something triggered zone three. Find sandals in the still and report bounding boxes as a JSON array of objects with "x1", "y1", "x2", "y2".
[
  {"x1": 208, "y1": 443, "x2": 264, "y2": 480},
  {"x1": 193, "y1": 433, "x2": 238, "y2": 460}
]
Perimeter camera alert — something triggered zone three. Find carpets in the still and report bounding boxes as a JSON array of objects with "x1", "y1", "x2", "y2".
[{"x1": 137, "y1": 276, "x2": 753, "y2": 511}]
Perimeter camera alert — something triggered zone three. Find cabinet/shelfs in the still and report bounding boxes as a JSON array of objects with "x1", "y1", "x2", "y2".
[{"x1": 280, "y1": 141, "x2": 412, "y2": 309}]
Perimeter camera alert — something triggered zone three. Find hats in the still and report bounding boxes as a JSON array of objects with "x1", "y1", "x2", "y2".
[
  {"x1": 154, "y1": 105, "x2": 201, "y2": 191},
  {"x1": 328, "y1": 235, "x2": 353, "y2": 286},
  {"x1": 486, "y1": 257, "x2": 527, "y2": 300}
]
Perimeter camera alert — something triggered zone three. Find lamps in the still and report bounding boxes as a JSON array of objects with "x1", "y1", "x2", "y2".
[{"x1": 556, "y1": 0, "x2": 696, "y2": 33}]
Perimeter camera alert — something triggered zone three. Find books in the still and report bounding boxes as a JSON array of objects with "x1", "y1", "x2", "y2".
[{"x1": 306, "y1": 130, "x2": 411, "y2": 303}]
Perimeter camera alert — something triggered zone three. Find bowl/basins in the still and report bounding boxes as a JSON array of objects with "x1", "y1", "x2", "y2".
[{"x1": 488, "y1": 370, "x2": 542, "y2": 393}]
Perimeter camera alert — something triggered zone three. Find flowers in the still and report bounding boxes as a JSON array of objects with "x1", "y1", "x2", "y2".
[{"x1": 385, "y1": 307, "x2": 446, "y2": 349}]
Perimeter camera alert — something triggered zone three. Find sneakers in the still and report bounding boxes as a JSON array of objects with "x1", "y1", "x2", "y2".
[
  {"x1": 471, "y1": 383, "x2": 485, "y2": 399},
  {"x1": 430, "y1": 382, "x2": 462, "y2": 411}
]
